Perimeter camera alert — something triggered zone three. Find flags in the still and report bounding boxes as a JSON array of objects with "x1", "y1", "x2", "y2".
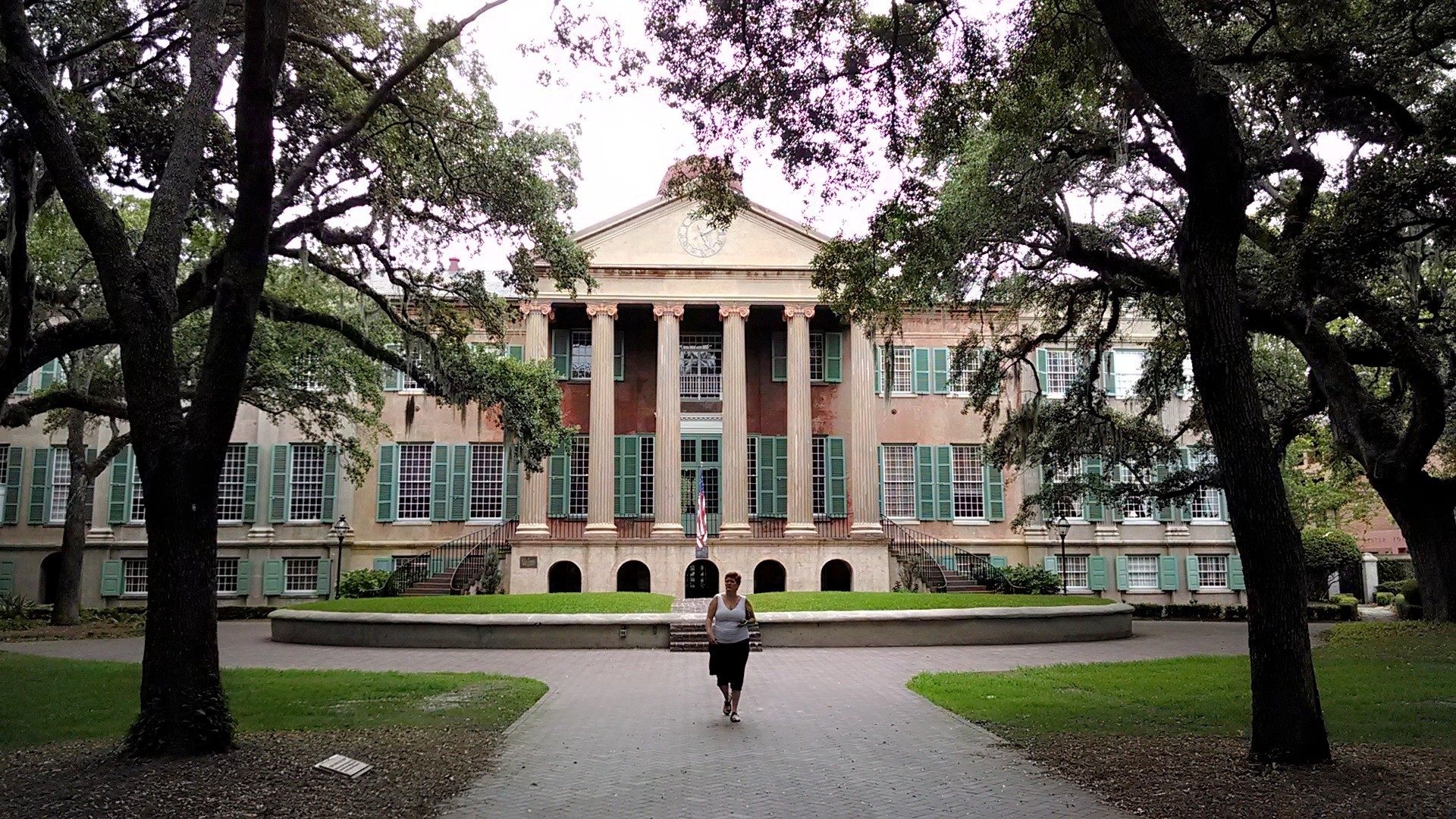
[{"x1": 696, "y1": 467, "x2": 708, "y2": 550}]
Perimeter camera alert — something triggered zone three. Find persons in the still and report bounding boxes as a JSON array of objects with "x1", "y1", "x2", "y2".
[{"x1": 706, "y1": 573, "x2": 756, "y2": 722}]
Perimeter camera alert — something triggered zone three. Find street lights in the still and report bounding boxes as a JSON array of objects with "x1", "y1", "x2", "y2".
[
  {"x1": 1055, "y1": 515, "x2": 1071, "y2": 594},
  {"x1": 334, "y1": 515, "x2": 350, "y2": 599}
]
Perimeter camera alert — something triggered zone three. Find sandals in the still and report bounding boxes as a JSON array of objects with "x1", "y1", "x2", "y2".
[
  {"x1": 723, "y1": 693, "x2": 733, "y2": 715},
  {"x1": 730, "y1": 712, "x2": 740, "y2": 723}
]
